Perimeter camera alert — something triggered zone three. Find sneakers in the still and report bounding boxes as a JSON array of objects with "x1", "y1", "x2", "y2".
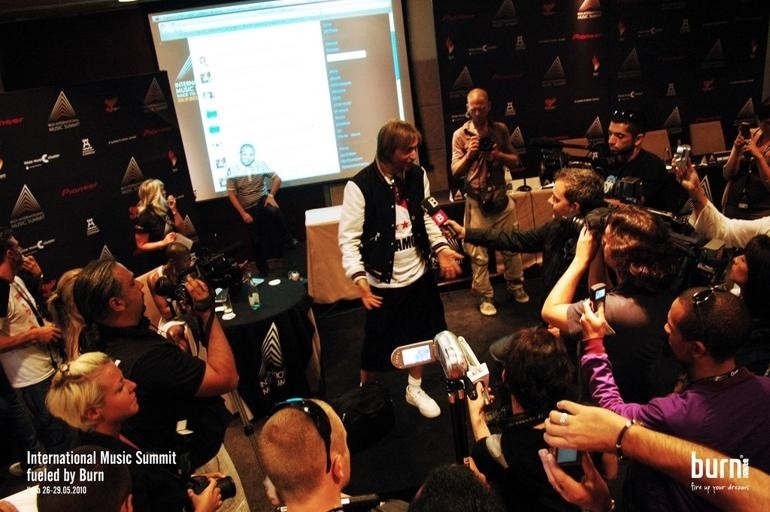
[
  {"x1": 406, "y1": 384, "x2": 442, "y2": 418},
  {"x1": 507, "y1": 286, "x2": 530, "y2": 304},
  {"x1": 480, "y1": 299, "x2": 497, "y2": 316}
]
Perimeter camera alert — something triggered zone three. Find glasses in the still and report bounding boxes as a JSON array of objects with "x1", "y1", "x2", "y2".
[
  {"x1": 275, "y1": 397, "x2": 331, "y2": 474},
  {"x1": 692, "y1": 282, "x2": 726, "y2": 346}
]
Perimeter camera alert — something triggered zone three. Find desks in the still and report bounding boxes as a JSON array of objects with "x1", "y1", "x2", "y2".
[
  {"x1": 304, "y1": 205, "x2": 362, "y2": 303},
  {"x1": 493, "y1": 177, "x2": 561, "y2": 274}
]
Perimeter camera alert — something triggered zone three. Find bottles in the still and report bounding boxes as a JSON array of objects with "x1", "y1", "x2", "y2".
[{"x1": 246, "y1": 271, "x2": 263, "y2": 311}]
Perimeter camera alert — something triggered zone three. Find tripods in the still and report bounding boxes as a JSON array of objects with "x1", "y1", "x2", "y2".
[{"x1": 183, "y1": 313, "x2": 265, "y2": 478}]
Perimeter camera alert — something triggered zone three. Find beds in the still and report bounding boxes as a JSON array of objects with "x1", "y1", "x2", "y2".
[{"x1": 214, "y1": 269, "x2": 304, "y2": 329}]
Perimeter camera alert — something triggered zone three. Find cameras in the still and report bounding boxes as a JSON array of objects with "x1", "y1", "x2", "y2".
[
  {"x1": 554, "y1": 444, "x2": 583, "y2": 470},
  {"x1": 390, "y1": 330, "x2": 489, "y2": 396},
  {"x1": 155, "y1": 233, "x2": 243, "y2": 316},
  {"x1": 178, "y1": 475, "x2": 236, "y2": 511},
  {"x1": 479, "y1": 136, "x2": 492, "y2": 151},
  {"x1": 676, "y1": 143, "x2": 691, "y2": 168}
]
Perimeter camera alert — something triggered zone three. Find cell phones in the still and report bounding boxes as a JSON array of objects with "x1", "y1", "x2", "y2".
[
  {"x1": 590, "y1": 282, "x2": 606, "y2": 313},
  {"x1": 740, "y1": 122, "x2": 751, "y2": 160}
]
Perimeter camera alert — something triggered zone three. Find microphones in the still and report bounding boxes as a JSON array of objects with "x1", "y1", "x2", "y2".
[{"x1": 420, "y1": 196, "x2": 457, "y2": 237}]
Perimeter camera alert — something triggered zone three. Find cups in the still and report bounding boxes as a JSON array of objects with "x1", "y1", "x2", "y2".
[{"x1": 221, "y1": 294, "x2": 233, "y2": 317}]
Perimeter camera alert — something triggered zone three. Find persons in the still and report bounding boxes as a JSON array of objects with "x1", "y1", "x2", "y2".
[
  {"x1": 336, "y1": 117, "x2": 463, "y2": 389},
  {"x1": 0, "y1": 226, "x2": 63, "y2": 460},
  {"x1": 48, "y1": 266, "x2": 88, "y2": 365},
  {"x1": 73, "y1": 262, "x2": 252, "y2": 512},
  {"x1": 128, "y1": 177, "x2": 184, "y2": 272},
  {"x1": 254, "y1": 397, "x2": 410, "y2": 512},
  {"x1": 409, "y1": 88, "x2": 770, "y2": 512},
  {"x1": 35, "y1": 349, "x2": 140, "y2": 512},
  {"x1": 147, "y1": 240, "x2": 199, "y2": 356},
  {"x1": 225, "y1": 143, "x2": 296, "y2": 280}
]
